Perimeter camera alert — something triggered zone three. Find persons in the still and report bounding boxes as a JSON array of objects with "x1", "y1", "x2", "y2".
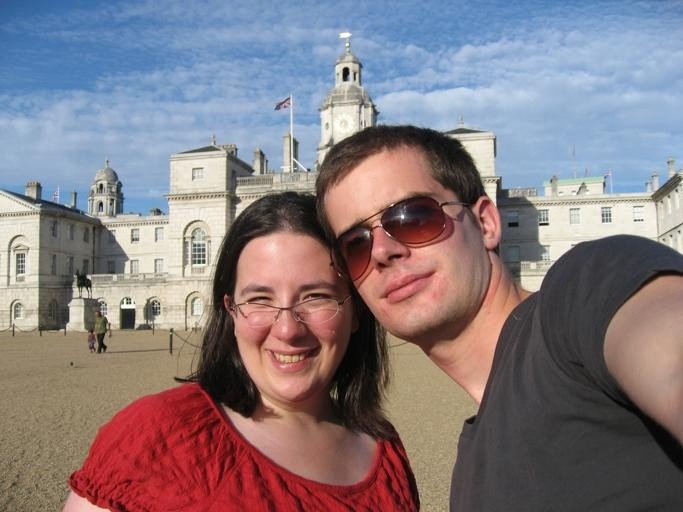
[
  {"x1": 314, "y1": 127, "x2": 681, "y2": 510},
  {"x1": 93, "y1": 309, "x2": 114, "y2": 352},
  {"x1": 60, "y1": 192, "x2": 421, "y2": 510},
  {"x1": 86, "y1": 328, "x2": 96, "y2": 350}
]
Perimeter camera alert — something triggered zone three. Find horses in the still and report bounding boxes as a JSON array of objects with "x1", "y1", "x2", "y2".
[{"x1": 75, "y1": 269, "x2": 92, "y2": 299}]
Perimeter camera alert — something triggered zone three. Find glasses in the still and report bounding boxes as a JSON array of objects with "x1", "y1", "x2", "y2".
[
  {"x1": 328, "y1": 195, "x2": 474, "y2": 282},
  {"x1": 224, "y1": 296, "x2": 352, "y2": 327}
]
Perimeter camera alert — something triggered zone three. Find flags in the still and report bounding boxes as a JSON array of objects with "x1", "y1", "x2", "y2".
[{"x1": 274, "y1": 96, "x2": 290, "y2": 111}]
